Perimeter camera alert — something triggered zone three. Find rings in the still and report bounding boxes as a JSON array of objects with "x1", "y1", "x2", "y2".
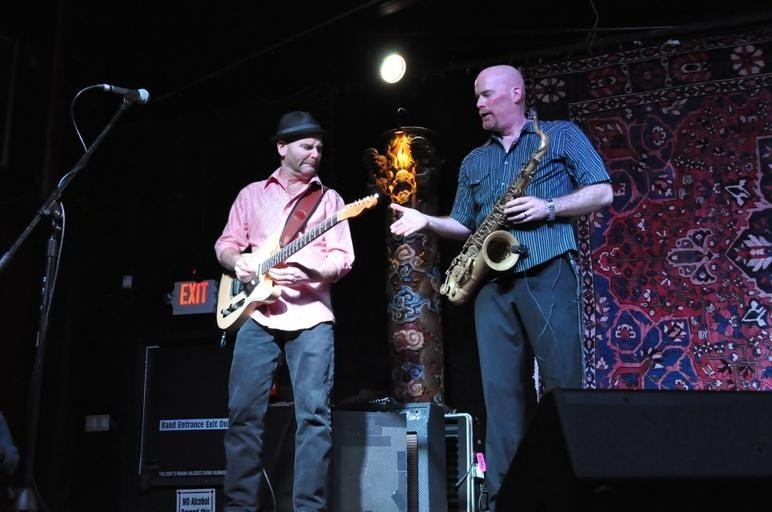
[{"x1": 522, "y1": 212, "x2": 527, "y2": 218}]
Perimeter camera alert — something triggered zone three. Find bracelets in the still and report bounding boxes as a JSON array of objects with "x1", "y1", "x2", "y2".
[{"x1": 544, "y1": 196, "x2": 556, "y2": 222}]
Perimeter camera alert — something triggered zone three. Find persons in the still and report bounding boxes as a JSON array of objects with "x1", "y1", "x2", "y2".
[
  {"x1": 387, "y1": 64, "x2": 614, "y2": 512},
  {"x1": 214, "y1": 111, "x2": 357, "y2": 511}
]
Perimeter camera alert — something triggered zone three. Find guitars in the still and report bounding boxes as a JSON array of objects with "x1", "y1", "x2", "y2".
[{"x1": 216, "y1": 193, "x2": 379, "y2": 334}]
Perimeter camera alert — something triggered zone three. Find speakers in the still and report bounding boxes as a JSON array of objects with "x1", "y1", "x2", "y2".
[
  {"x1": 137, "y1": 342, "x2": 242, "y2": 488},
  {"x1": 498, "y1": 390, "x2": 771, "y2": 509}
]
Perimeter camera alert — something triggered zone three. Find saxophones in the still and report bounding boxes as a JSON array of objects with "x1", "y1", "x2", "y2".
[{"x1": 440, "y1": 108, "x2": 549, "y2": 304}]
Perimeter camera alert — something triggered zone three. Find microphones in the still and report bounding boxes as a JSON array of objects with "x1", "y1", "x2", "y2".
[
  {"x1": 510, "y1": 244, "x2": 526, "y2": 256},
  {"x1": 104, "y1": 82, "x2": 152, "y2": 107}
]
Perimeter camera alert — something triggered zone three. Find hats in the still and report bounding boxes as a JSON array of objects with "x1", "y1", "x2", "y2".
[{"x1": 272, "y1": 110, "x2": 327, "y2": 145}]
